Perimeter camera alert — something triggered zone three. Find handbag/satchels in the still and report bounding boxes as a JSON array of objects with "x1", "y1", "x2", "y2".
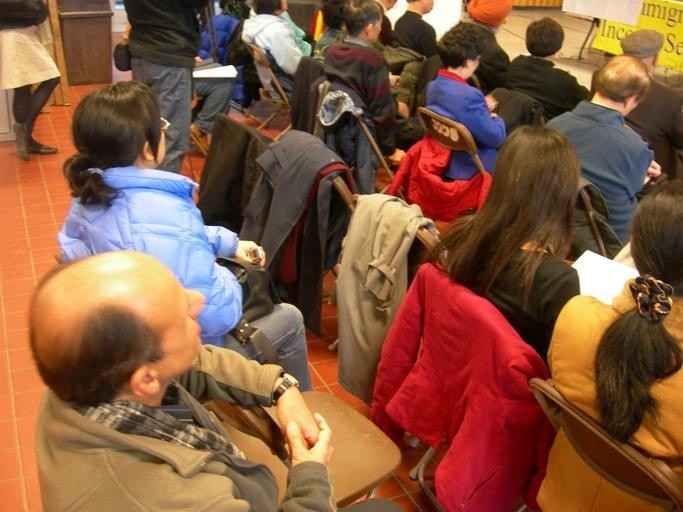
[
  {"x1": 216, "y1": 256, "x2": 282, "y2": 346},
  {"x1": 114, "y1": 42, "x2": 132, "y2": 71}
]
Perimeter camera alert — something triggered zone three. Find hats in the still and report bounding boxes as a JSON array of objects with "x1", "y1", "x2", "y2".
[
  {"x1": 467, "y1": 1, "x2": 513, "y2": 26},
  {"x1": 621, "y1": 29, "x2": 665, "y2": 59},
  {"x1": 526, "y1": 17, "x2": 564, "y2": 56}
]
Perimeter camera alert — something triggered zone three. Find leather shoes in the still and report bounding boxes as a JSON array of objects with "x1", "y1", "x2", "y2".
[
  {"x1": 28, "y1": 144, "x2": 57, "y2": 154},
  {"x1": 13, "y1": 123, "x2": 30, "y2": 160},
  {"x1": 189, "y1": 124, "x2": 209, "y2": 156}
]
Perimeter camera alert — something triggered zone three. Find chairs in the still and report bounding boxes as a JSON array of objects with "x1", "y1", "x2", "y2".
[{"x1": 114, "y1": 0, "x2": 682, "y2": 512}]
